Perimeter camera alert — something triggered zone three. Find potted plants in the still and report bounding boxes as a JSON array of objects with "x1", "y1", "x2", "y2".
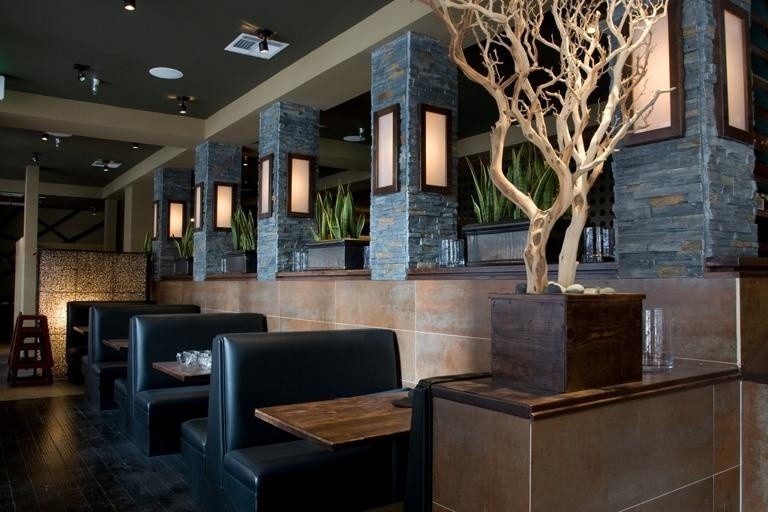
[
  {"x1": 167, "y1": 219, "x2": 198, "y2": 277},
  {"x1": 420, "y1": 0, "x2": 678, "y2": 395},
  {"x1": 220, "y1": 203, "x2": 259, "y2": 272},
  {"x1": 299, "y1": 177, "x2": 371, "y2": 270}
]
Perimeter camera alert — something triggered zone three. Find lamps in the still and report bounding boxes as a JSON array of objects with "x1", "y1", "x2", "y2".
[
  {"x1": 73, "y1": 62, "x2": 88, "y2": 83},
  {"x1": 255, "y1": 27, "x2": 275, "y2": 56},
  {"x1": 102, "y1": 160, "x2": 111, "y2": 173},
  {"x1": 176, "y1": 95, "x2": 192, "y2": 115}
]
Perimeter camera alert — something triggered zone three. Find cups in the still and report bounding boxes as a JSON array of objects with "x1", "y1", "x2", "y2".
[
  {"x1": 642, "y1": 307, "x2": 674, "y2": 373},
  {"x1": 439, "y1": 238, "x2": 466, "y2": 268},
  {"x1": 363, "y1": 245, "x2": 370, "y2": 268},
  {"x1": 581, "y1": 225, "x2": 614, "y2": 264},
  {"x1": 176, "y1": 350, "x2": 212, "y2": 370},
  {"x1": 220, "y1": 256, "x2": 226, "y2": 273},
  {"x1": 292, "y1": 250, "x2": 309, "y2": 271}
]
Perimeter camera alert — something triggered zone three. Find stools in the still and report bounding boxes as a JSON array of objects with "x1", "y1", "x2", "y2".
[{"x1": 4, "y1": 311, "x2": 57, "y2": 389}]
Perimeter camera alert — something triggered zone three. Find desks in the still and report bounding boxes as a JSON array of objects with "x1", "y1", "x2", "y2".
[{"x1": 255, "y1": 385, "x2": 419, "y2": 508}]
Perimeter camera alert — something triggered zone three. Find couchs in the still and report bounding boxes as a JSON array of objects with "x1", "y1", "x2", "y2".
[{"x1": 64, "y1": 297, "x2": 406, "y2": 512}]
